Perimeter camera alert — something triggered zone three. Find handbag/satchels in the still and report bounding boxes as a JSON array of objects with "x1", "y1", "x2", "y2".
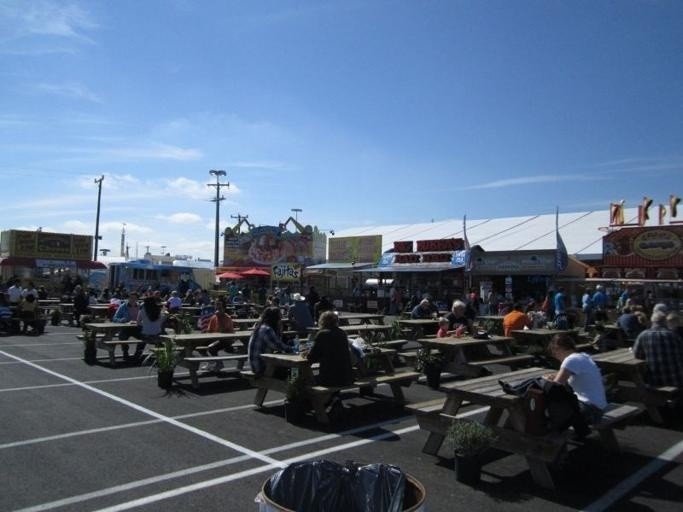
[{"x1": 526, "y1": 388, "x2": 551, "y2": 434}]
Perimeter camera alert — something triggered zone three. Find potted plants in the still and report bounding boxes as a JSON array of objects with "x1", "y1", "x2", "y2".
[
  {"x1": 143, "y1": 340, "x2": 179, "y2": 389},
  {"x1": 450, "y1": 421, "x2": 502, "y2": 483},
  {"x1": 420, "y1": 358, "x2": 443, "y2": 390}
]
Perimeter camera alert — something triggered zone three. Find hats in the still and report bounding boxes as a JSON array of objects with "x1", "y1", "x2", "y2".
[
  {"x1": 291, "y1": 292, "x2": 305, "y2": 301},
  {"x1": 26, "y1": 294, "x2": 34, "y2": 302}
]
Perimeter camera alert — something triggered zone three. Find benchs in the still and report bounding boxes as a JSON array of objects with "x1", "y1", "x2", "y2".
[
  {"x1": 405, "y1": 396, "x2": 473, "y2": 416},
  {"x1": 546, "y1": 401, "x2": 647, "y2": 445},
  {"x1": 647, "y1": 385, "x2": 683, "y2": 402},
  {"x1": 574, "y1": 343, "x2": 592, "y2": 349},
  {"x1": 75, "y1": 333, "x2": 119, "y2": 339},
  {"x1": 238, "y1": 370, "x2": 257, "y2": 381},
  {"x1": 149, "y1": 343, "x2": 243, "y2": 353},
  {"x1": 183, "y1": 355, "x2": 248, "y2": 362},
  {"x1": 371, "y1": 339, "x2": 408, "y2": 347},
  {"x1": 468, "y1": 354, "x2": 534, "y2": 366},
  {"x1": 398, "y1": 349, "x2": 440, "y2": 358},
  {"x1": 309, "y1": 369, "x2": 422, "y2": 394},
  {"x1": 102, "y1": 339, "x2": 144, "y2": 345}
]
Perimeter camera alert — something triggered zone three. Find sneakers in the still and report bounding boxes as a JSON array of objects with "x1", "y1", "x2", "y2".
[
  {"x1": 124, "y1": 352, "x2": 128, "y2": 360},
  {"x1": 210, "y1": 363, "x2": 217, "y2": 372},
  {"x1": 327, "y1": 400, "x2": 343, "y2": 417},
  {"x1": 201, "y1": 362, "x2": 210, "y2": 370},
  {"x1": 135, "y1": 352, "x2": 142, "y2": 359}
]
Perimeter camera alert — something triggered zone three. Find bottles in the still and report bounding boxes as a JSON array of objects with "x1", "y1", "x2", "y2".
[{"x1": 294, "y1": 335, "x2": 300, "y2": 354}]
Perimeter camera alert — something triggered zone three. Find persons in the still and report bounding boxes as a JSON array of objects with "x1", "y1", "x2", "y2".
[
  {"x1": 502, "y1": 296, "x2": 537, "y2": 338},
  {"x1": 665, "y1": 311, "x2": 683, "y2": 336},
  {"x1": 633, "y1": 310, "x2": 683, "y2": 389},
  {"x1": 0, "y1": 273, "x2": 50, "y2": 337},
  {"x1": 541, "y1": 332, "x2": 608, "y2": 433},
  {"x1": 644, "y1": 289, "x2": 658, "y2": 317},
  {"x1": 217, "y1": 294, "x2": 226, "y2": 314},
  {"x1": 447, "y1": 299, "x2": 470, "y2": 333},
  {"x1": 400, "y1": 291, "x2": 440, "y2": 321},
  {"x1": 614, "y1": 285, "x2": 649, "y2": 346},
  {"x1": 351, "y1": 280, "x2": 367, "y2": 312},
  {"x1": 228, "y1": 281, "x2": 253, "y2": 306},
  {"x1": 581, "y1": 286, "x2": 594, "y2": 333},
  {"x1": 136, "y1": 296, "x2": 165, "y2": 341},
  {"x1": 264, "y1": 294, "x2": 281, "y2": 310},
  {"x1": 138, "y1": 283, "x2": 164, "y2": 301},
  {"x1": 468, "y1": 285, "x2": 481, "y2": 306},
  {"x1": 167, "y1": 290, "x2": 182, "y2": 315},
  {"x1": 112, "y1": 292, "x2": 146, "y2": 365},
  {"x1": 653, "y1": 302, "x2": 670, "y2": 314},
  {"x1": 247, "y1": 307, "x2": 306, "y2": 379},
  {"x1": 121, "y1": 289, "x2": 129, "y2": 300},
  {"x1": 591, "y1": 284, "x2": 611, "y2": 322},
  {"x1": 588, "y1": 320, "x2": 610, "y2": 354},
  {"x1": 201, "y1": 302, "x2": 236, "y2": 373},
  {"x1": 112, "y1": 287, "x2": 122, "y2": 299},
  {"x1": 288, "y1": 292, "x2": 316, "y2": 345},
  {"x1": 307, "y1": 285, "x2": 319, "y2": 316},
  {"x1": 301, "y1": 310, "x2": 354, "y2": 420},
  {"x1": 544, "y1": 291, "x2": 555, "y2": 322},
  {"x1": 436, "y1": 316, "x2": 452, "y2": 339},
  {"x1": 554, "y1": 287, "x2": 568, "y2": 321},
  {"x1": 59, "y1": 274, "x2": 112, "y2": 327},
  {"x1": 485, "y1": 288, "x2": 509, "y2": 317},
  {"x1": 182, "y1": 288, "x2": 212, "y2": 307},
  {"x1": 313, "y1": 294, "x2": 336, "y2": 326}
]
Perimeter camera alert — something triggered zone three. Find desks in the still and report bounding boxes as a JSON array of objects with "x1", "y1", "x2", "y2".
[
  {"x1": 232, "y1": 317, "x2": 289, "y2": 331},
  {"x1": 339, "y1": 324, "x2": 393, "y2": 340},
  {"x1": 252, "y1": 348, "x2": 404, "y2": 425},
  {"x1": 84, "y1": 322, "x2": 143, "y2": 366},
  {"x1": 510, "y1": 328, "x2": 579, "y2": 363},
  {"x1": 395, "y1": 319, "x2": 440, "y2": 337},
  {"x1": 338, "y1": 314, "x2": 385, "y2": 324},
  {"x1": 0, "y1": 294, "x2": 260, "y2": 321},
  {"x1": 475, "y1": 315, "x2": 504, "y2": 334},
  {"x1": 158, "y1": 332, "x2": 252, "y2": 390},
  {"x1": 417, "y1": 335, "x2": 517, "y2": 374},
  {"x1": 421, "y1": 366, "x2": 566, "y2": 488},
  {"x1": 591, "y1": 347, "x2": 664, "y2": 425}
]
